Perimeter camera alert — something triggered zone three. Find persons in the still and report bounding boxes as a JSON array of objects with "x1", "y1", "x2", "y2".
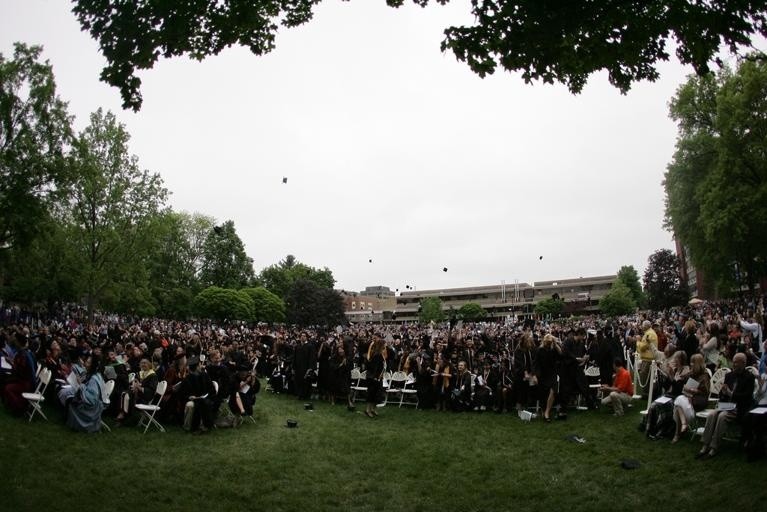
[{"x1": 1, "y1": 295, "x2": 766, "y2": 459}]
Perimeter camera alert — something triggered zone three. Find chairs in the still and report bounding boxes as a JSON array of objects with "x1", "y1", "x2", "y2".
[
  {"x1": 0, "y1": 346, "x2": 259, "y2": 433},
  {"x1": 527, "y1": 360, "x2": 765, "y2": 447},
  {"x1": 264, "y1": 347, "x2": 492, "y2": 408}
]
